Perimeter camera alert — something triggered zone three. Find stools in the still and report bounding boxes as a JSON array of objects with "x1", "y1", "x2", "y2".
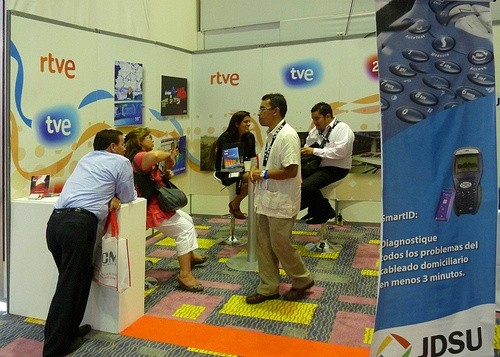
[
  {"x1": 305, "y1": 173, "x2": 349, "y2": 253},
  {"x1": 212, "y1": 170, "x2": 248, "y2": 247}
]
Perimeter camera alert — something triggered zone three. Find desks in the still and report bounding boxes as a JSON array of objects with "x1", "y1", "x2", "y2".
[{"x1": 9, "y1": 192, "x2": 147, "y2": 335}]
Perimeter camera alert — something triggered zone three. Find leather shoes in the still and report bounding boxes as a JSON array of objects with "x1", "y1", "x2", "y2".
[
  {"x1": 301, "y1": 213, "x2": 312, "y2": 220},
  {"x1": 72, "y1": 324, "x2": 92, "y2": 338},
  {"x1": 282, "y1": 280, "x2": 314, "y2": 299},
  {"x1": 50, "y1": 337, "x2": 85, "y2": 357},
  {"x1": 246, "y1": 291, "x2": 280, "y2": 304},
  {"x1": 306, "y1": 210, "x2": 336, "y2": 224}
]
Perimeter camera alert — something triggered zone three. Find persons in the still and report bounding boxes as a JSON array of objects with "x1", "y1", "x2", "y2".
[
  {"x1": 299, "y1": 101, "x2": 355, "y2": 224},
  {"x1": 246, "y1": 94, "x2": 315, "y2": 305},
  {"x1": 213, "y1": 111, "x2": 257, "y2": 219},
  {"x1": 122, "y1": 128, "x2": 208, "y2": 291},
  {"x1": 42, "y1": 129, "x2": 136, "y2": 357}
]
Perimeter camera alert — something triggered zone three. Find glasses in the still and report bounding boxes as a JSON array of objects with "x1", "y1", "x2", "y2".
[
  {"x1": 258, "y1": 108, "x2": 274, "y2": 113},
  {"x1": 145, "y1": 135, "x2": 152, "y2": 140}
]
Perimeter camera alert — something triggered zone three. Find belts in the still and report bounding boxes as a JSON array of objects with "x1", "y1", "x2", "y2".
[{"x1": 54, "y1": 208, "x2": 98, "y2": 224}]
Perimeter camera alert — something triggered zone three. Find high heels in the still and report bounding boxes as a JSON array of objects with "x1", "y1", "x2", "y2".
[
  {"x1": 229, "y1": 202, "x2": 245, "y2": 219},
  {"x1": 189, "y1": 254, "x2": 208, "y2": 267},
  {"x1": 177, "y1": 274, "x2": 204, "y2": 292}
]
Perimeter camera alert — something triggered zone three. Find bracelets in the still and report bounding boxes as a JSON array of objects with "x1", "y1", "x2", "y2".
[{"x1": 264, "y1": 170, "x2": 269, "y2": 179}]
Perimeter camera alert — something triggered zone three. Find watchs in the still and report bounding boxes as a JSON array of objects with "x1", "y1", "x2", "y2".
[{"x1": 260, "y1": 170, "x2": 265, "y2": 179}]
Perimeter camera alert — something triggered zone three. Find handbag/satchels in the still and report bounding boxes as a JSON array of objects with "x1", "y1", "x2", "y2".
[
  {"x1": 300, "y1": 142, "x2": 321, "y2": 179},
  {"x1": 155, "y1": 175, "x2": 187, "y2": 212},
  {"x1": 93, "y1": 209, "x2": 131, "y2": 292}
]
